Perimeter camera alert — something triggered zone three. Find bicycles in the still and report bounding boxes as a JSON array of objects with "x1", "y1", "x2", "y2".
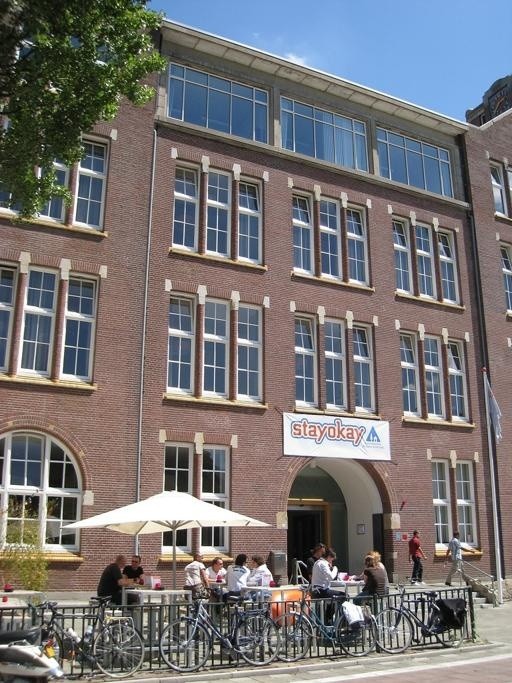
[
  {"x1": 267, "y1": 584, "x2": 379, "y2": 663},
  {"x1": 32, "y1": 594, "x2": 146, "y2": 676},
  {"x1": 371, "y1": 585, "x2": 468, "y2": 653},
  {"x1": 160, "y1": 590, "x2": 281, "y2": 672}
]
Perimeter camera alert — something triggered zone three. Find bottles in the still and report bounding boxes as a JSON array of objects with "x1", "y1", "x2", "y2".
[
  {"x1": 63, "y1": 628, "x2": 81, "y2": 643},
  {"x1": 82, "y1": 625, "x2": 94, "y2": 642}
]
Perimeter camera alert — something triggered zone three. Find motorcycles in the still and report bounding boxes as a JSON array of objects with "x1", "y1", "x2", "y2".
[{"x1": 0, "y1": 627, "x2": 65, "y2": 682}]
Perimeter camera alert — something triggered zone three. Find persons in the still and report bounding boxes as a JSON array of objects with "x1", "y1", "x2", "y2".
[
  {"x1": 443, "y1": 530, "x2": 476, "y2": 586},
  {"x1": 405, "y1": 525, "x2": 429, "y2": 588},
  {"x1": 95, "y1": 540, "x2": 394, "y2": 621}
]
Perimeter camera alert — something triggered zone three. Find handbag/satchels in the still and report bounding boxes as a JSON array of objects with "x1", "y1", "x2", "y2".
[{"x1": 439, "y1": 599, "x2": 466, "y2": 627}]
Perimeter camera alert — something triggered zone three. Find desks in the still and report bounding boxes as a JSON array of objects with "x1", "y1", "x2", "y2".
[{"x1": 0, "y1": 577, "x2": 390, "y2": 644}]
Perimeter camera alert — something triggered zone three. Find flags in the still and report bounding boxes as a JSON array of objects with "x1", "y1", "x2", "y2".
[{"x1": 485, "y1": 374, "x2": 503, "y2": 446}]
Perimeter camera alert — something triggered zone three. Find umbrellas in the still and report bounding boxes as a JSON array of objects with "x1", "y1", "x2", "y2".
[{"x1": 58, "y1": 489, "x2": 275, "y2": 592}]
